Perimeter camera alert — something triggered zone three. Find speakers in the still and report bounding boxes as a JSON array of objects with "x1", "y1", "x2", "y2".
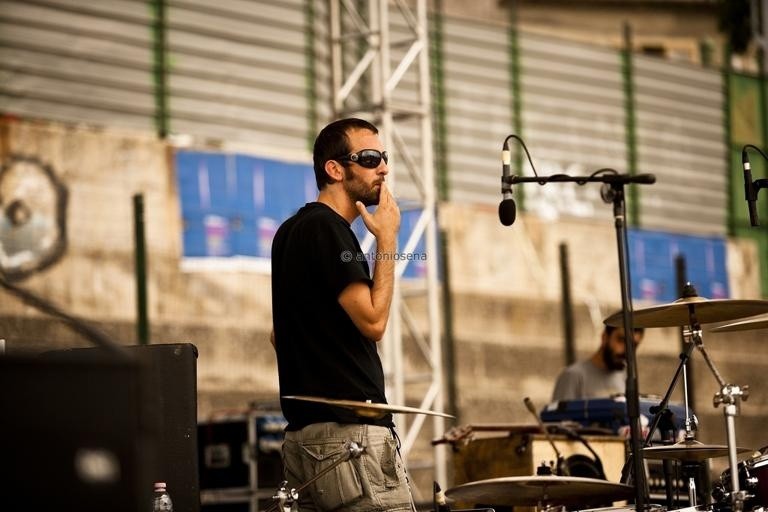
[{"x1": 0, "y1": 342, "x2": 201, "y2": 512}]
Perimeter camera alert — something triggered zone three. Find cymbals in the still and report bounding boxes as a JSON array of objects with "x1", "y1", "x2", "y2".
[
  {"x1": 604, "y1": 298, "x2": 768, "y2": 330},
  {"x1": 712, "y1": 318, "x2": 768, "y2": 332},
  {"x1": 639, "y1": 440, "x2": 753, "y2": 461},
  {"x1": 284, "y1": 396, "x2": 455, "y2": 418},
  {"x1": 445, "y1": 473, "x2": 640, "y2": 507}
]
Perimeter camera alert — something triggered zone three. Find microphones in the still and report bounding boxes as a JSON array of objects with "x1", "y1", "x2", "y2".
[
  {"x1": 523, "y1": 397, "x2": 573, "y2": 476},
  {"x1": 499, "y1": 144, "x2": 516, "y2": 226},
  {"x1": 742, "y1": 151, "x2": 761, "y2": 227}
]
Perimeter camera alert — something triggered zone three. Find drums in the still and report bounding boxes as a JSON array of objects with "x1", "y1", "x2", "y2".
[{"x1": 720, "y1": 452, "x2": 767, "y2": 510}]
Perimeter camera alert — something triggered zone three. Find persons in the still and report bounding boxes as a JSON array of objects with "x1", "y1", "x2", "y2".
[
  {"x1": 273, "y1": 117, "x2": 417, "y2": 510},
  {"x1": 553, "y1": 319, "x2": 645, "y2": 434}
]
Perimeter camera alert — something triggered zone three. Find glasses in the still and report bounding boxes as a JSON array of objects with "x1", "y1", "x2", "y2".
[{"x1": 336, "y1": 149, "x2": 387, "y2": 168}]
